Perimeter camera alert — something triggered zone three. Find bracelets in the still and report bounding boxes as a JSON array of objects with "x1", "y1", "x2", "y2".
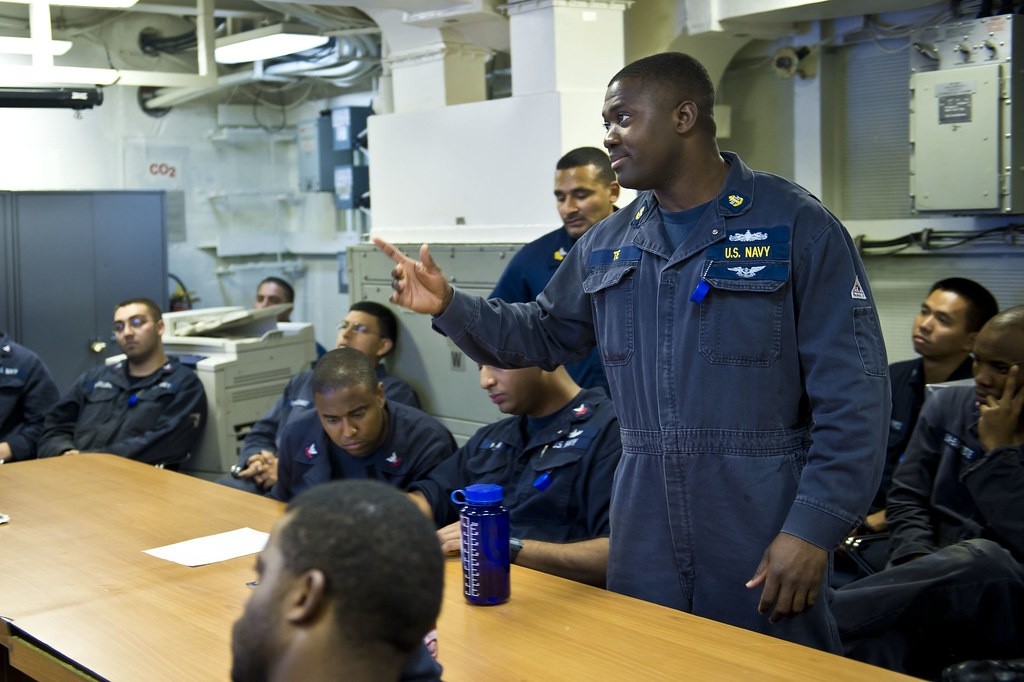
[{"x1": 510, "y1": 537, "x2": 521, "y2": 562}]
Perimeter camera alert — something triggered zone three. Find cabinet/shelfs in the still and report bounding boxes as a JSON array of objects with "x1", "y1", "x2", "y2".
[{"x1": 0, "y1": 190, "x2": 169, "y2": 402}]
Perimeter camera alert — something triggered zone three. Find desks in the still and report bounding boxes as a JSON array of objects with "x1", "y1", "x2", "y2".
[{"x1": 0, "y1": 452, "x2": 933, "y2": 682}]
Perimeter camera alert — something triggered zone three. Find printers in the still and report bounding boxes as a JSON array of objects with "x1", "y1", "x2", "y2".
[{"x1": 104, "y1": 302, "x2": 318, "y2": 484}]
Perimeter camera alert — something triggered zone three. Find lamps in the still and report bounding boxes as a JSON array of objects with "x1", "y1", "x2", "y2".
[{"x1": 213, "y1": 22, "x2": 328, "y2": 65}]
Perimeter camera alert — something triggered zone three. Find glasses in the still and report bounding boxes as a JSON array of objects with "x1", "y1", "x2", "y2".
[
  {"x1": 113, "y1": 317, "x2": 156, "y2": 332},
  {"x1": 337, "y1": 320, "x2": 383, "y2": 336}
]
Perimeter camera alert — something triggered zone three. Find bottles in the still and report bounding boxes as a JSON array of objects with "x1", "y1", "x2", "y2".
[{"x1": 451, "y1": 484, "x2": 510, "y2": 606}]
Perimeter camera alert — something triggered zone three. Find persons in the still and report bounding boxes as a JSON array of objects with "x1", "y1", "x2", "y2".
[
  {"x1": 255, "y1": 277, "x2": 326, "y2": 367},
  {"x1": 265, "y1": 348, "x2": 458, "y2": 491},
  {"x1": 374, "y1": 53, "x2": 891, "y2": 653},
  {"x1": 401, "y1": 363, "x2": 622, "y2": 586},
  {"x1": 484, "y1": 147, "x2": 619, "y2": 401},
  {"x1": 833, "y1": 304, "x2": 1024, "y2": 674},
  {"x1": 217, "y1": 301, "x2": 422, "y2": 492},
  {"x1": 847, "y1": 277, "x2": 999, "y2": 537},
  {"x1": 231, "y1": 480, "x2": 445, "y2": 682},
  {"x1": 0, "y1": 331, "x2": 61, "y2": 463},
  {"x1": 38, "y1": 299, "x2": 208, "y2": 470}
]
